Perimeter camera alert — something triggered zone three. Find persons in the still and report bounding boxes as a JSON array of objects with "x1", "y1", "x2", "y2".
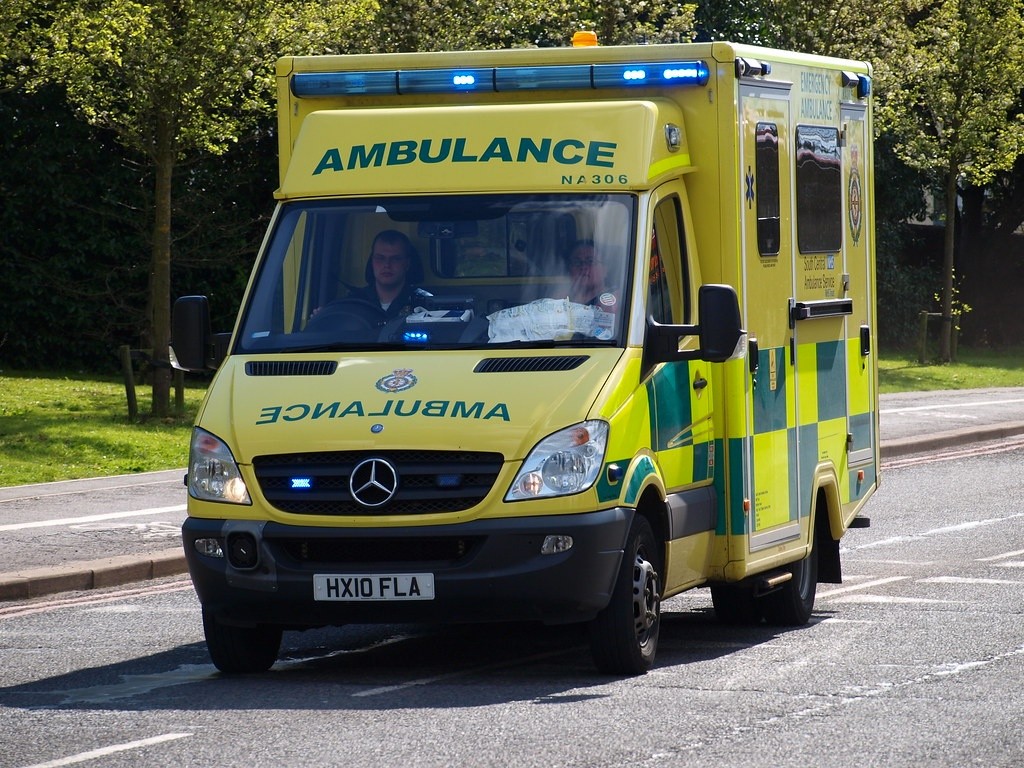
[
  {"x1": 550, "y1": 238, "x2": 621, "y2": 313},
  {"x1": 310, "y1": 230, "x2": 434, "y2": 329}
]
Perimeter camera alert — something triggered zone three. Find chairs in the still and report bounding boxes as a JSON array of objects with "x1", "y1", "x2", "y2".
[
  {"x1": 366, "y1": 243, "x2": 425, "y2": 286},
  {"x1": 602, "y1": 245, "x2": 624, "y2": 289}
]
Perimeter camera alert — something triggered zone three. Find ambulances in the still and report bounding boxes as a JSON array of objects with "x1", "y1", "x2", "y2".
[{"x1": 165, "y1": 31, "x2": 881, "y2": 674}]
